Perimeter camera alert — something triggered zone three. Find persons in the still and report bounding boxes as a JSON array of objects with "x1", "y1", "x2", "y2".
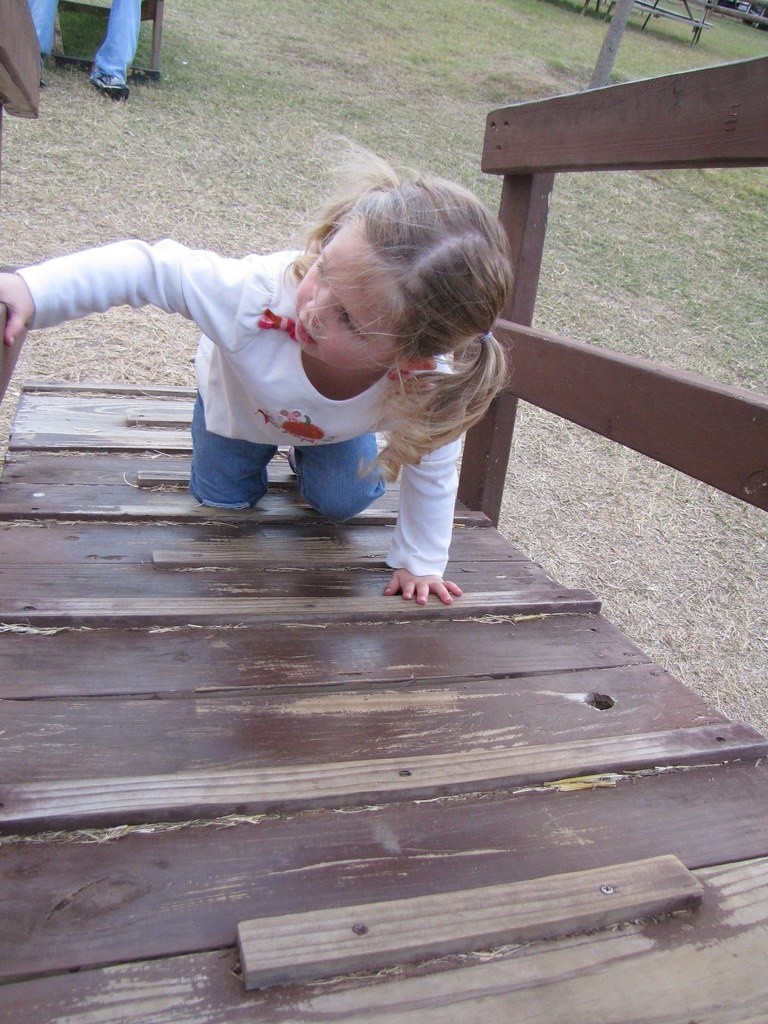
[
  {"x1": 27, "y1": 0, "x2": 145, "y2": 101},
  {"x1": 0, "y1": 161, "x2": 517, "y2": 605}
]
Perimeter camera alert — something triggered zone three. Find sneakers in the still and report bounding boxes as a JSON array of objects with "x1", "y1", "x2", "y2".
[
  {"x1": 40, "y1": 58, "x2": 48, "y2": 87},
  {"x1": 90, "y1": 66, "x2": 129, "y2": 100}
]
